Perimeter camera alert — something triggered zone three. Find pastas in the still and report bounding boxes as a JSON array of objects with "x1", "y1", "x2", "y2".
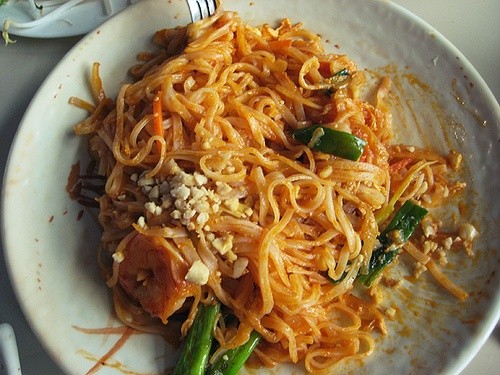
[{"x1": 67, "y1": 8, "x2": 478, "y2": 374}]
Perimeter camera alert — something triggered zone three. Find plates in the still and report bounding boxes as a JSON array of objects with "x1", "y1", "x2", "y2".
[
  {"x1": 0, "y1": 0, "x2": 135, "y2": 39},
  {"x1": 1, "y1": 0, "x2": 499, "y2": 375}
]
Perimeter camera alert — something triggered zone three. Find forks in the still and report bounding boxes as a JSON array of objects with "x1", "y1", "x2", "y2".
[{"x1": 187, "y1": 0, "x2": 217, "y2": 21}]
